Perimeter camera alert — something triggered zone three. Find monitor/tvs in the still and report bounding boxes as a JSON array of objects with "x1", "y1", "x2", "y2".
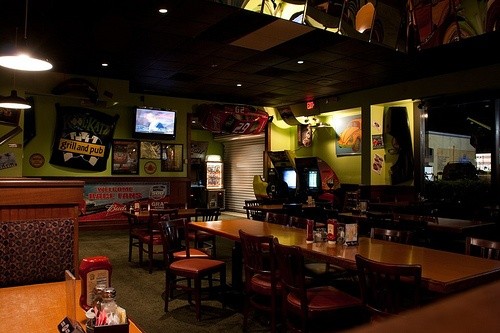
[
  {"x1": 281, "y1": 170, "x2": 297, "y2": 189},
  {"x1": 134, "y1": 107, "x2": 176, "y2": 140},
  {"x1": 306, "y1": 169, "x2": 320, "y2": 189}
]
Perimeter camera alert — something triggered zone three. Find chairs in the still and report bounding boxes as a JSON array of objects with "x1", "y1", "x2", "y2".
[{"x1": 126, "y1": 198, "x2": 500, "y2": 333}]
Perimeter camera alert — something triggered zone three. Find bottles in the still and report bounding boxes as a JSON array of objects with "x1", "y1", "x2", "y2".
[
  {"x1": 93, "y1": 284, "x2": 106, "y2": 317},
  {"x1": 101, "y1": 287, "x2": 117, "y2": 315},
  {"x1": 316, "y1": 229, "x2": 327, "y2": 241},
  {"x1": 338, "y1": 227, "x2": 345, "y2": 242},
  {"x1": 79, "y1": 256, "x2": 113, "y2": 312},
  {"x1": 130, "y1": 206, "x2": 133, "y2": 214},
  {"x1": 306, "y1": 220, "x2": 314, "y2": 244},
  {"x1": 133, "y1": 201, "x2": 140, "y2": 215},
  {"x1": 327, "y1": 219, "x2": 337, "y2": 244}
]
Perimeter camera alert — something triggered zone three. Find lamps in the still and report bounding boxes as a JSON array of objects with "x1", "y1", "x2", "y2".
[
  {"x1": 0, "y1": 72, "x2": 31, "y2": 109},
  {"x1": 0, "y1": 0, "x2": 52, "y2": 72}
]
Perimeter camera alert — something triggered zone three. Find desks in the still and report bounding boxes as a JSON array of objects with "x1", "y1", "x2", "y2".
[
  {"x1": 243, "y1": 204, "x2": 315, "y2": 223},
  {"x1": 122, "y1": 209, "x2": 200, "y2": 265},
  {"x1": 292, "y1": 238, "x2": 500, "y2": 294},
  {"x1": 339, "y1": 212, "x2": 500, "y2": 244},
  {"x1": 188, "y1": 217, "x2": 336, "y2": 283},
  {"x1": 0, "y1": 278, "x2": 142, "y2": 333}
]
